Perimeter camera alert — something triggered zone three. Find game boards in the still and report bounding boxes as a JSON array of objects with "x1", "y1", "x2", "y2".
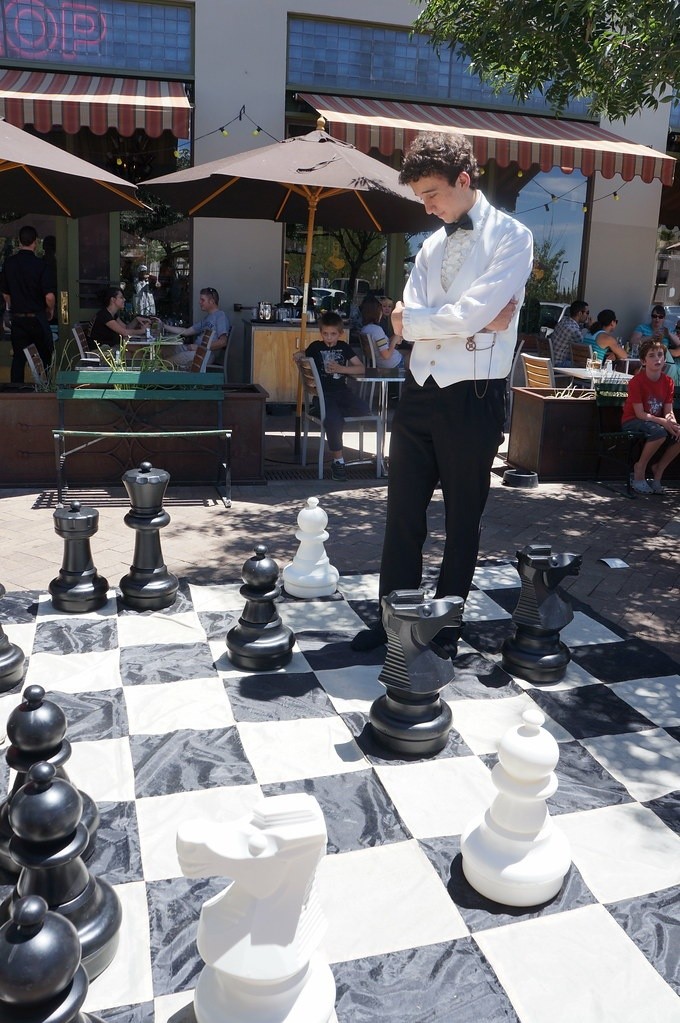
[{"x1": 0, "y1": 555, "x2": 680, "y2": 1023}]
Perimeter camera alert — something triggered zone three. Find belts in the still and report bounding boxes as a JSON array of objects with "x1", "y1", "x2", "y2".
[{"x1": 12, "y1": 312, "x2": 49, "y2": 319}]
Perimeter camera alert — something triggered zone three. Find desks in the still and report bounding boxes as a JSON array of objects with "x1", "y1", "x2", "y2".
[
  {"x1": 345, "y1": 367, "x2": 406, "y2": 478},
  {"x1": 553, "y1": 367, "x2": 634, "y2": 390},
  {"x1": 618, "y1": 350, "x2": 641, "y2": 374},
  {"x1": 123, "y1": 335, "x2": 183, "y2": 360}
]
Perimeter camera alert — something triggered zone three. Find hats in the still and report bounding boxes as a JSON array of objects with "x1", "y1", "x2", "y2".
[{"x1": 138, "y1": 265, "x2": 148, "y2": 273}]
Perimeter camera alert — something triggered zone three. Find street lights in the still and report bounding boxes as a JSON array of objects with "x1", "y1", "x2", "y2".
[{"x1": 558, "y1": 261, "x2": 568, "y2": 292}]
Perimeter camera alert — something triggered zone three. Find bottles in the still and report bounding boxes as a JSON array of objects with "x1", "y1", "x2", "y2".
[
  {"x1": 251, "y1": 308, "x2": 258, "y2": 320},
  {"x1": 145, "y1": 328, "x2": 150, "y2": 338},
  {"x1": 625, "y1": 340, "x2": 629, "y2": 353}
]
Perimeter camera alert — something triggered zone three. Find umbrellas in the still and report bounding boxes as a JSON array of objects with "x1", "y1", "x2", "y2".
[
  {"x1": 0, "y1": 119, "x2": 154, "y2": 220},
  {"x1": 136, "y1": 116, "x2": 444, "y2": 456}
]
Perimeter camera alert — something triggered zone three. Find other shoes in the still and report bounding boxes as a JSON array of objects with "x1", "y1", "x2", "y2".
[
  {"x1": 308, "y1": 408, "x2": 318, "y2": 421},
  {"x1": 331, "y1": 459, "x2": 346, "y2": 481}
]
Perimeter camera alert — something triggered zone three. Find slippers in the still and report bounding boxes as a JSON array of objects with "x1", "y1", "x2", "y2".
[{"x1": 631, "y1": 472, "x2": 666, "y2": 495}]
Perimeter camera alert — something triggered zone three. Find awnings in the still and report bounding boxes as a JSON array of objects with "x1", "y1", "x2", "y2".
[
  {"x1": 0, "y1": 68, "x2": 192, "y2": 140},
  {"x1": 294, "y1": 92, "x2": 678, "y2": 186}
]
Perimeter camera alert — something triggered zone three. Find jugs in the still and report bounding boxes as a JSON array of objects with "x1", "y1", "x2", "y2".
[{"x1": 258, "y1": 301, "x2": 317, "y2": 324}]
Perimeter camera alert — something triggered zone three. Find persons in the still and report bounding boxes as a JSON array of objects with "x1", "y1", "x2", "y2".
[
  {"x1": 552, "y1": 301, "x2": 680, "y2": 371},
  {"x1": 351, "y1": 133, "x2": 533, "y2": 658},
  {"x1": 0, "y1": 225, "x2": 57, "y2": 384},
  {"x1": 89, "y1": 287, "x2": 151, "y2": 367},
  {"x1": 123, "y1": 260, "x2": 176, "y2": 318},
  {"x1": 150, "y1": 287, "x2": 229, "y2": 365},
  {"x1": 622, "y1": 338, "x2": 680, "y2": 493},
  {"x1": 293, "y1": 311, "x2": 365, "y2": 480},
  {"x1": 358, "y1": 294, "x2": 407, "y2": 406}
]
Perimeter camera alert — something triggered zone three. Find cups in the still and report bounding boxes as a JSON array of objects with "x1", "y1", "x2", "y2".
[
  {"x1": 323, "y1": 351, "x2": 335, "y2": 373},
  {"x1": 616, "y1": 337, "x2": 623, "y2": 347},
  {"x1": 588, "y1": 358, "x2": 614, "y2": 376},
  {"x1": 632, "y1": 344, "x2": 639, "y2": 359}
]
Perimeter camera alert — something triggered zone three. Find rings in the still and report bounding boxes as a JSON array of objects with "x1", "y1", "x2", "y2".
[{"x1": 512, "y1": 313, "x2": 514, "y2": 317}]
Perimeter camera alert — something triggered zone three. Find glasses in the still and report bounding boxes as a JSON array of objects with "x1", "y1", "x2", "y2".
[
  {"x1": 208, "y1": 287, "x2": 219, "y2": 305},
  {"x1": 583, "y1": 310, "x2": 590, "y2": 316},
  {"x1": 651, "y1": 314, "x2": 665, "y2": 319},
  {"x1": 613, "y1": 320, "x2": 618, "y2": 325}
]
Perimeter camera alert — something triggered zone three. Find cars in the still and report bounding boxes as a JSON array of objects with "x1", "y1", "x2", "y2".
[
  {"x1": 522, "y1": 301, "x2": 572, "y2": 323},
  {"x1": 284, "y1": 286, "x2": 322, "y2": 305},
  {"x1": 665, "y1": 305, "x2": 680, "y2": 320},
  {"x1": 312, "y1": 288, "x2": 347, "y2": 307}
]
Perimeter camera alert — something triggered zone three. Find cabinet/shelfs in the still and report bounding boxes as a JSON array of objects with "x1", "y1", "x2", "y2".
[{"x1": 245, "y1": 322, "x2": 349, "y2": 403}]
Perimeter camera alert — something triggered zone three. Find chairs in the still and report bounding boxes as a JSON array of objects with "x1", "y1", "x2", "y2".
[
  {"x1": 520, "y1": 353, "x2": 556, "y2": 388},
  {"x1": 23, "y1": 325, "x2": 235, "y2": 385},
  {"x1": 296, "y1": 356, "x2": 383, "y2": 479},
  {"x1": 505, "y1": 340, "x2": 524, "y2": 419},
  {"x1": 359, "y1": 331, "x2": 402, "y2": 410},
  {"x1": 570, "y1": 341, "x2": 593, "y2": 390},
  {"x1": 535, "y1": 336, "x2": 554, "y2": 365}
]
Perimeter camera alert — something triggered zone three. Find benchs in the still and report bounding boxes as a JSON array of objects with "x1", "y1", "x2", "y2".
[
  {"x1": 596, "y1": 382, "x2": 680, "y2": 498},
  {"x1": 52, "y1": 372, "x2": 234, "y2": 508}
]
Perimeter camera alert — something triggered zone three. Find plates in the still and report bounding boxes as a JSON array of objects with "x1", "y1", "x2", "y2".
[{"x1": 285, "y1": 318, "x2": 303, "y2": 323}]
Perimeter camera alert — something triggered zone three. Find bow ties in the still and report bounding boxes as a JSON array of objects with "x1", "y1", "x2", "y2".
[{"x1": 444, "y1": 213, "x2": 473, "y2": 236}]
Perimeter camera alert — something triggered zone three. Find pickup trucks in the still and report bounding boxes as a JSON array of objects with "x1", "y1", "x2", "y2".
[{"x1": 327, "y1": 278, "x2": 386, "y2": 307}]
